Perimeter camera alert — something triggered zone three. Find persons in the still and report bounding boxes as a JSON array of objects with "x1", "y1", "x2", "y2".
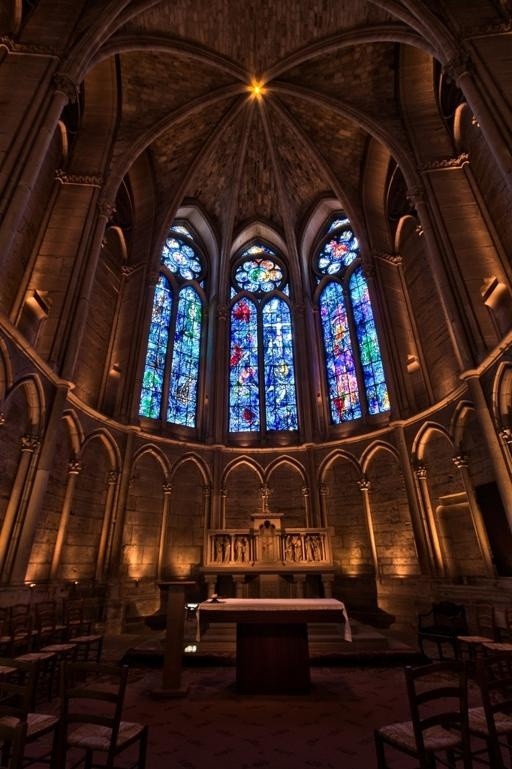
[
  {"x1": 292, "y1": 535, "x2": 303, "y2": 562},
  {"x1": 215, "y1": 536, "x2": 223, "y2": 564},
  {"x1": 223, "y1": 535, "x2": 231, "y2": 563},
  {"x1": 235, "y1": 537, "x2": 243, "y2": 563},
  {"x1": 243, "y1": 537, "x2": 250, "y2": 562},
  {"x1": 285, "y1": 535, "x2": 294, "y2": 563},
  {"x1": 305, "y1": 535, "x2": 315, "y2": 562},
  {"x1": 311, "y1": 535, "x2": 322, "y2": 562}
]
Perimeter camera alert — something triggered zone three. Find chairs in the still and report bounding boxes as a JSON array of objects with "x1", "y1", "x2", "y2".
[
  {"x1": 0, "y1": 598, "x2": 148, "y2": 768},
  {"x1": 374, "y1": 601, "x2": 512, "y2": 769}
]
